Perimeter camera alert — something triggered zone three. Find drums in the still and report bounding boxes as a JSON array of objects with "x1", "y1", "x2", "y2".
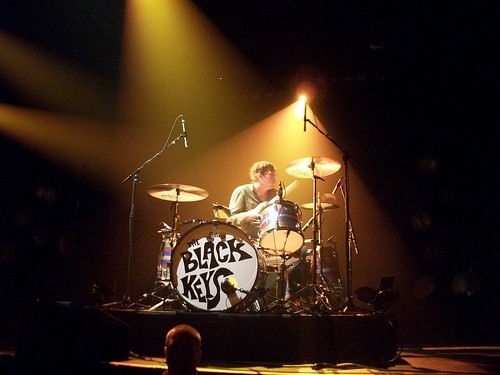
[
  {"x1": 170, "y1": 221, "x2": 268, "y2": 314},
  {"x1": 258, "y1": 200, "x2": 305, "y2": 256},
  {"x1": 178, "y1": 219, "x2": 206, "y2": 236},
  {"x1": 304, "y1": 239, "x2": 343, "y2": 291}
]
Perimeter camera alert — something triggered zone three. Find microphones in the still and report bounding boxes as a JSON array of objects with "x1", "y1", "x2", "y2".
[
  {"x1": 332, "y1": 175, "x2": 344, "y2": 193},
  {"x1": 304, "y1": 105, "x2": 306, "y2": 131},
  {"x1": 181, "y1": 119, "x2": 189, "y2": 147},
  {"x1": 278, "y1": 183, "x2": 282, "y2": 199},
  {"x1": 221, "y1": 280, "x2": 232, "y2": 291}
]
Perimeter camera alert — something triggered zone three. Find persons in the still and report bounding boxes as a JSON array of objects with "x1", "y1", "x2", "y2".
[
  {"x1": 163, "y1": 326, "x2": 204, "y2": 375},
  {"x1": 228, "y1": 160, "x2": 306, "y2": 299}
]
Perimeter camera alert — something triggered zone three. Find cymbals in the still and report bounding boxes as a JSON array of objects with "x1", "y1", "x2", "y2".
[
  {"x1": 300, "y1": 202, "x2": 340, "y2": 210},
  {"x1": 147, "y1": 183, "x2": 210, "y2": 202},
  {"x1": 284, "y1": 156, "x2": 342, "y2": 178}
]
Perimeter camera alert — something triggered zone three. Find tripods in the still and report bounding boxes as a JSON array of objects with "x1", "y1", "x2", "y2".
[{"x1": 273, "y1": 177, "x2": 345, "y2": 316}]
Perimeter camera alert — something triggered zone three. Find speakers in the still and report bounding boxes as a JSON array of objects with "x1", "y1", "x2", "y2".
[{"x1": 56, "y1": 306, "x2": 134, "y2": 363}]
